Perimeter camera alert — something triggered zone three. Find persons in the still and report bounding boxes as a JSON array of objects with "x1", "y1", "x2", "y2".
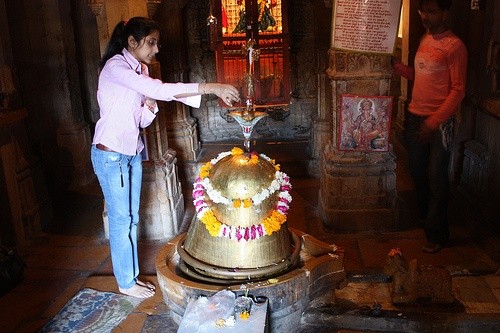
[
  {"x1": 391, "y1": 0, "x2": 468, "y2": 252},
  {"x1": 90, "y1": 16, "x2": 240, "y2": 297}
]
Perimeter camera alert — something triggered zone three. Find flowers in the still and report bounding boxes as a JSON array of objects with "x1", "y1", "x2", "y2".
[{"x1": 190, "y1": 150, "x2": 293, "y2": 242}]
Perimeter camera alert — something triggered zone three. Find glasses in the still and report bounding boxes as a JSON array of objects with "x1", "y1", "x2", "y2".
[
  {"x1": 142, "y1": 38, "x2": 162, "y2": 49},
  {"x1": 417, "y1": 7, "x2": 442, "y2": 15}
]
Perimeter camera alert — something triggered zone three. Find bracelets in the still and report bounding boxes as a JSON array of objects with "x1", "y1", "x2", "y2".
[{"x1": 202, "y1": 82, "x2": 206, "y2": 94}]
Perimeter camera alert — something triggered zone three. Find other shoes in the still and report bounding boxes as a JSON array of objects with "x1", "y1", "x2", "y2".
[{"x1": 422, "y1": 237, "x2": 442, "y2": 253}]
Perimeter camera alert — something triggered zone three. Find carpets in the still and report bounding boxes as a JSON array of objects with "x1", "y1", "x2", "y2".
[{"x1": 39, "y1": 288, "x2": 142, "y2": 333}]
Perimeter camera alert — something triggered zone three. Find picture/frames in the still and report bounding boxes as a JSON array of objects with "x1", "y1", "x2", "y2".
[{"x1": 337, "y1": 93, "x2": 394, "y2": 153}]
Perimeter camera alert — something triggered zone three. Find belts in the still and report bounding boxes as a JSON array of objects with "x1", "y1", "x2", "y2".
[{"x1": 96, "y1": 143, "x2": 115, "y2": 152}]
